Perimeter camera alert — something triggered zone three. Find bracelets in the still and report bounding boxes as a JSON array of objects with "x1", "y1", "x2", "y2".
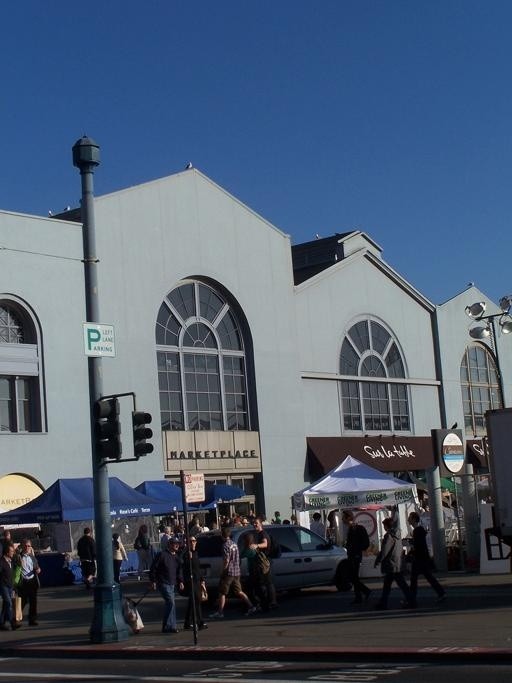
[{"x1": 257, "y1": 544, "x2": 259, "y2": 548}]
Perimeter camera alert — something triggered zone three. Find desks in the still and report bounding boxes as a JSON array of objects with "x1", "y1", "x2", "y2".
[{"x1": 465, "y1": 295, "x2": 512, "y2": 410}]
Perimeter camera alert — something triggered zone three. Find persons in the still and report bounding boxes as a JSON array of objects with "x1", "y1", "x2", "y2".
[
  {"x1": 183, "y1": 535, "x2": 208, "y2": 631},
  {"x1": 374, "y1": 518, "x2": 416, "y2": 611},
  {"x1": 249, "y1": 516, "x2": 272, "y2": 616},
  {"x1": 399, "y1": 512, "x2": 444, "y2": 606},
  {"x1": 77, "y1": 527, "x2": 96, "y2": 590},
  {"x1": 135, "y1": 524, "x2": 154, "y2": 580},
  {"x1": 271, "y1": 512, "x2": 299, "y2": 533},
  {"x1": 160, "y1": 511, "x2": 256, "y2": 556},
  {"x1": 310, "y1": 513, "x2": 325, "y2": 543},
  {"x1": 342, "y1": 511, "x2": 370, "y2": 603},
  {"x1": 112, "y1": 533, "x2": 128, "y2": 584},
  {"x1": 149, "y1": 538, "x2": 184, "y2": 633},
  {"x1": 208, "y1": 528, "x2": 256, "y2": 618},
  {"x1": 1, "y1": 530, "x2": 41, "y2": 633}
]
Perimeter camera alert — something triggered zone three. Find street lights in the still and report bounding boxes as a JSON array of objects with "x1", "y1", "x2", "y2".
[
  {"x1": 200, "y1": 580, "x2": 208, "y2": 601},
  {"x1": 11, "y1": 595, "x2": 23, "y2": 622},
  {"x1": 258, "y1": 549, "x2": 270, "y2": 573}
]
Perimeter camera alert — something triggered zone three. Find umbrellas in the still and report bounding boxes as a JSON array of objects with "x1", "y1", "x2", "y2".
[{"x1": 162, "y1": 623, "x2": 207, "y2": 633}]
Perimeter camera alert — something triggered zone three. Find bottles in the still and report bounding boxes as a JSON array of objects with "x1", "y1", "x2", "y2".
[{"x1": 169, "y1": 537, "x2": 181, "y2": 544}]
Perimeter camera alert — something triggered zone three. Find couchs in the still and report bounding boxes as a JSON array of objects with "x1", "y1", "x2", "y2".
[
  {"x1": 93, "y1": 397, "x2": 122, "y2": 460},
  {"x1": 132, "y1": 411, "x2": 154, "y2": 458}
]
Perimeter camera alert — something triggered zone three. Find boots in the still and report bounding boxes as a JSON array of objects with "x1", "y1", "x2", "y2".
[{"x1": 178, "y1": 524, "x2": 354, "y2": 601}]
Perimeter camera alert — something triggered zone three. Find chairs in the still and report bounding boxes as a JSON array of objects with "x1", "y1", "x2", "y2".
[{"x1": 208, "y1": 612, "x2": 224, "y2": 619}]
